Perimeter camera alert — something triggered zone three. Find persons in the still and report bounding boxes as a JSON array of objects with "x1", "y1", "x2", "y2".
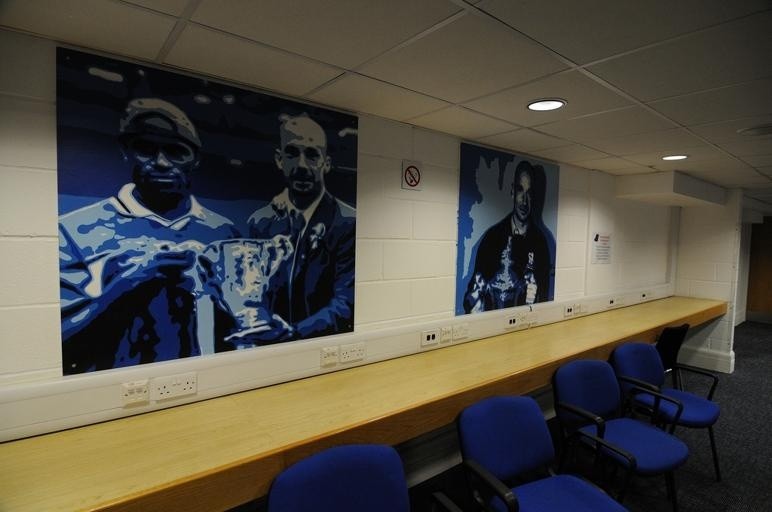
[
  {"x1": 241, "y1": 110, "x2": 356, "y2": 350},
  {"x1": 57, "y1": 93, "x2": 241, "y2": 377},
  {"x1": 461, "y1": 157, "x2": 551, "y2": 313}
]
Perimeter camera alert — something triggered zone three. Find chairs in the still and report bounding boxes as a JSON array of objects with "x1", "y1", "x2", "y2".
[
  {"x1": 608, "y1": 340, "x2": 726, "y2": 483},
  {"x1": 652, "y1": 320, "x2": 692, "y2": 388},
  {"x1": 267, "y1": 442, "x2": 411, "y2": 511},
  {"x1": 549, "y1": 357, "x2": 692, "y2": 511},
  {"x1": 457, "y1": 395, "x2": 637, "y2": 511}
]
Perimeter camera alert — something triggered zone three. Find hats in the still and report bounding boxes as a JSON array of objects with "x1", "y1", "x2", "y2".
[{"x1": 119, "y1": 96, "x2": 203, "y2": 149}]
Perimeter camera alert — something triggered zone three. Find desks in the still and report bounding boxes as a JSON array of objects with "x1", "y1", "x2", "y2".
[{"x1": 1, "y1": 295, "x2": 728, "y2": 511}]
